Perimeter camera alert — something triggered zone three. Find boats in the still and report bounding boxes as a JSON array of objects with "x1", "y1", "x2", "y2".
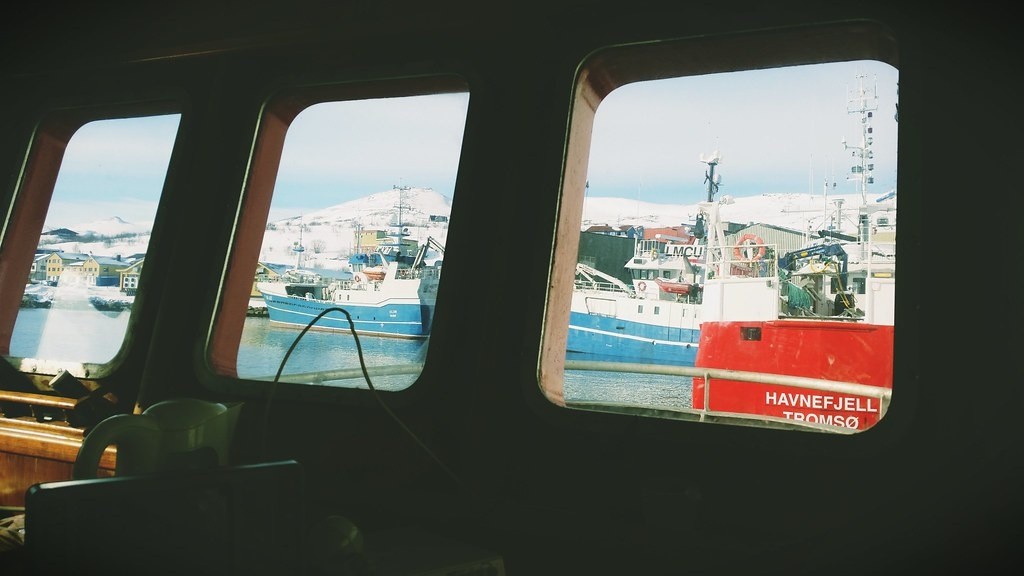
[
  {"x1": 254, "y1": 69, "x2": 896, "y2": 430},
  {"x1": 655, "y1": 277, "x2": 693, "y2": 294}
]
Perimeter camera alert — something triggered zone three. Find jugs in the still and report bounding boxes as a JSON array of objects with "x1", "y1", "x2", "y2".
[{"x1": 73, "y1": 399, "x2": 245, "y2": 480}]
